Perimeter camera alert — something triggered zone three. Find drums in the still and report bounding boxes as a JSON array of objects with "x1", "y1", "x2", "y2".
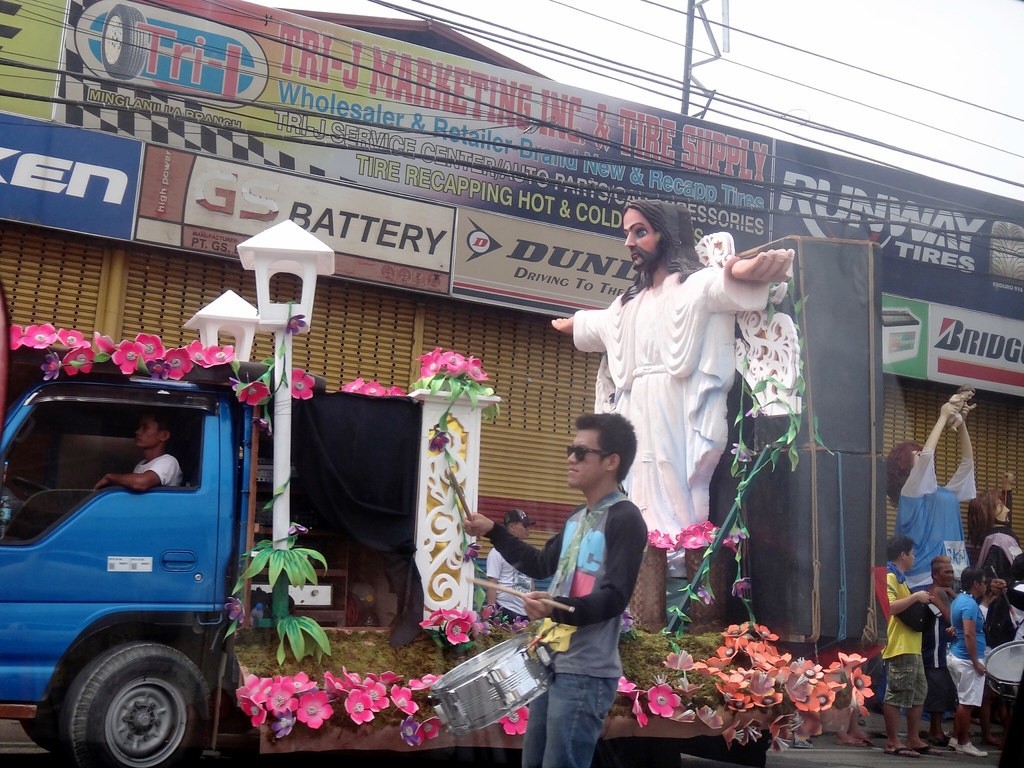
[
  {"x1": 984, "y1": 640, "x2": 1024, "y2": 701},
  {"x1": 426, "y1": 632, "x2": 556, "y2": 738}
]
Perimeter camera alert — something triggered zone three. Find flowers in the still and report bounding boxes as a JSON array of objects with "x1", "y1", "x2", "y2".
[{"x1": 11, "y1": 318, "x2": 873, "y2": 745}]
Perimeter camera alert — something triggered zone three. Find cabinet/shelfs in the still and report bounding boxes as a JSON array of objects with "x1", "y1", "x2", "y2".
[{"x1": 250, "y1": 523, "x2": 350, "y2": 627}]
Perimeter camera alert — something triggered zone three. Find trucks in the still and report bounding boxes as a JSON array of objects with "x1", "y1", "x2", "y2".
[{"x1": 0, "y1": 336, "x2": 792, "y2": 768}]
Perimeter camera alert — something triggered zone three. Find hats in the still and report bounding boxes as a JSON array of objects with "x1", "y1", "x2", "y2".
[{"x1": 504, "y1": 509, "x2": 536, "y2": 526}]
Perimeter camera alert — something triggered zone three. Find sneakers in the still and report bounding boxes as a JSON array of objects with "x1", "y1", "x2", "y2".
[
  {"x1": 949, "y1": 737, "x2": 958, "y2": 748},
  {"x1": 956, "y1": 743, "x2": 989, "y2": 757}
]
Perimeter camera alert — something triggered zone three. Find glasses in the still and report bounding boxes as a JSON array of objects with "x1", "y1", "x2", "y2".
[{"x1": 567, "y1": 446, "x2": 611, "y2": 461}]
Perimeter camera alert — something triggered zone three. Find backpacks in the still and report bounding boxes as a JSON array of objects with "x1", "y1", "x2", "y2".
[{"x1": 982, "y1": 580, "x2": 1024, "y2": 648}]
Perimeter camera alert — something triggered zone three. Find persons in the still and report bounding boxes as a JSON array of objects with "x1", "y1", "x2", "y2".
[
  {"x1": 884, "y1": 404, "x2": 976, "y2": 593},
  {"x1": 462, "y1": 413, "x2": 650, "y2": 768},
  {"x1": 970, "y1": 490, "x2": 1022, "y2": 603},
  {"x1": 778, "y1": 536, "x2": 1023, "y2": 768},
  {"x1": 946, "y1": 385, "x2": 978, "y2": 434},
  {"x1": 485, "y1": 509, "x2": 536, "y2": 625},
  {"x1": 93, "y1": 418, "x2": 181, "y2": 490},
  {"x1": 555, "y1": 199, "x2": 794, "y2": 635}
]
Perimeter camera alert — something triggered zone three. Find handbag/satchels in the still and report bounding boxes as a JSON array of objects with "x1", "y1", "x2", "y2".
[{"x1": 896, "y1": 601, "x2": 943, "y2": 633}]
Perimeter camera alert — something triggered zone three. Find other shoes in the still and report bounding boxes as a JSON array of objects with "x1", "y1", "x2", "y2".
[
  {"x1": 793, "y1": 735, "x2": 814, "y2": 749},
  {"x1": 834, "y1": 733, "x2": 874, "y2": 747},
  {"x1": 983, "y1": 736, "x2": 1005, "y2": 747},
  {"x1": 928, "y1": 736, "x2": 950, "y2": 747}
]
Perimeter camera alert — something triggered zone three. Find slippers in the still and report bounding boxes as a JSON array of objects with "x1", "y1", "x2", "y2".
[
  {"x1": 914, "y1": 745, "x2": 938, "y2": 755},
  {"x1": 883, "y1": 747, "x2": 920, "y2": 758}
]
